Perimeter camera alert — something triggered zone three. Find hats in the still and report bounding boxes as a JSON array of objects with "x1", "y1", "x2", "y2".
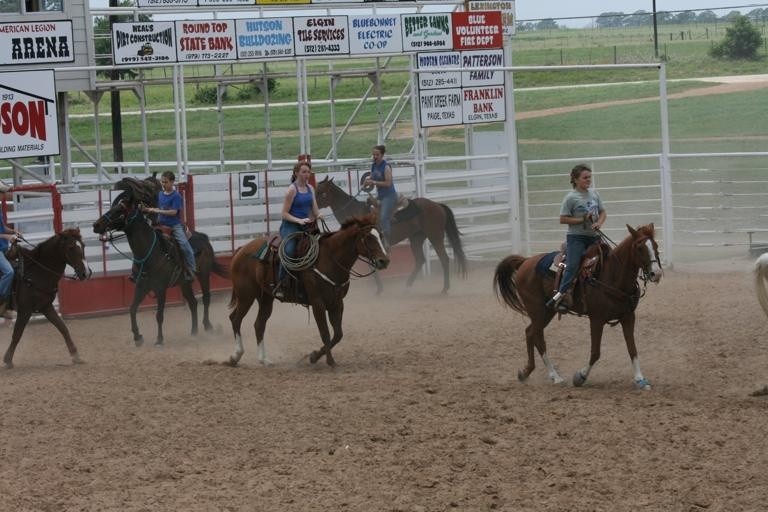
[{"x1": 0, "y1": 181, "x2": 10, "y2": 192}]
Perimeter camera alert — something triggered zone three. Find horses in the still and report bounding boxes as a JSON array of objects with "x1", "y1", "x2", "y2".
[
  {"x1": 315, "y1": 174, "x2": 468, "y2": 295},
  {"x1": 228, "y1": 216, "x2": 390, "y2": 367},
  {"x1": 493, "y1": 222, "x2": 665, "y2": 391},
  {"x1": 92, "y1": 197, "x2": 232, "y2": 346},
  {"x1": 0, "y1": 227, "x2": 92, "y2": 369},
  {"x1": 748, "y1": 252, "x2": 768, "y2": 396}
]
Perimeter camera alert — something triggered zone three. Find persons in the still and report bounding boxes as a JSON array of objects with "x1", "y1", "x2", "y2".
[
  {"x1": 0, "y1": 195, "x2": 28, "y2": 320},
  {"x1": 273, "y1": 161, "x2": 326, "y2": 300},
  {"x1": 554, "y1": 162, "x2": 606, "y2": 314},
  {"x1": 128, "y1": 171, "x2": 196, "y2": 283},
  {"x1": 363, "y1": 144, "x2": 398, "y2": 248}
]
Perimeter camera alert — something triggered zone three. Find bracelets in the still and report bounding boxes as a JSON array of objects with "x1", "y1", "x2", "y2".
[{"x1": 372, "y1": 180, "x2": 377, "y2": 186}]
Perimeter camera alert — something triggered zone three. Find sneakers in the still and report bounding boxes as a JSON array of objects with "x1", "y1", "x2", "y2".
[
  {"x1": 558, "y1": 304, "x2": 567, "y2": 312},
  {"x1": 128, "y1": 273, "x2": 136, "y2": 280},
  {"x1": 275, "y1": 287, "x2": 287, "y2": 298}
]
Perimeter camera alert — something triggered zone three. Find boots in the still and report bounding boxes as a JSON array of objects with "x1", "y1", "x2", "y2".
[{"x1": 0, "y1": 302, "x2": 13, "y2": 319}]
